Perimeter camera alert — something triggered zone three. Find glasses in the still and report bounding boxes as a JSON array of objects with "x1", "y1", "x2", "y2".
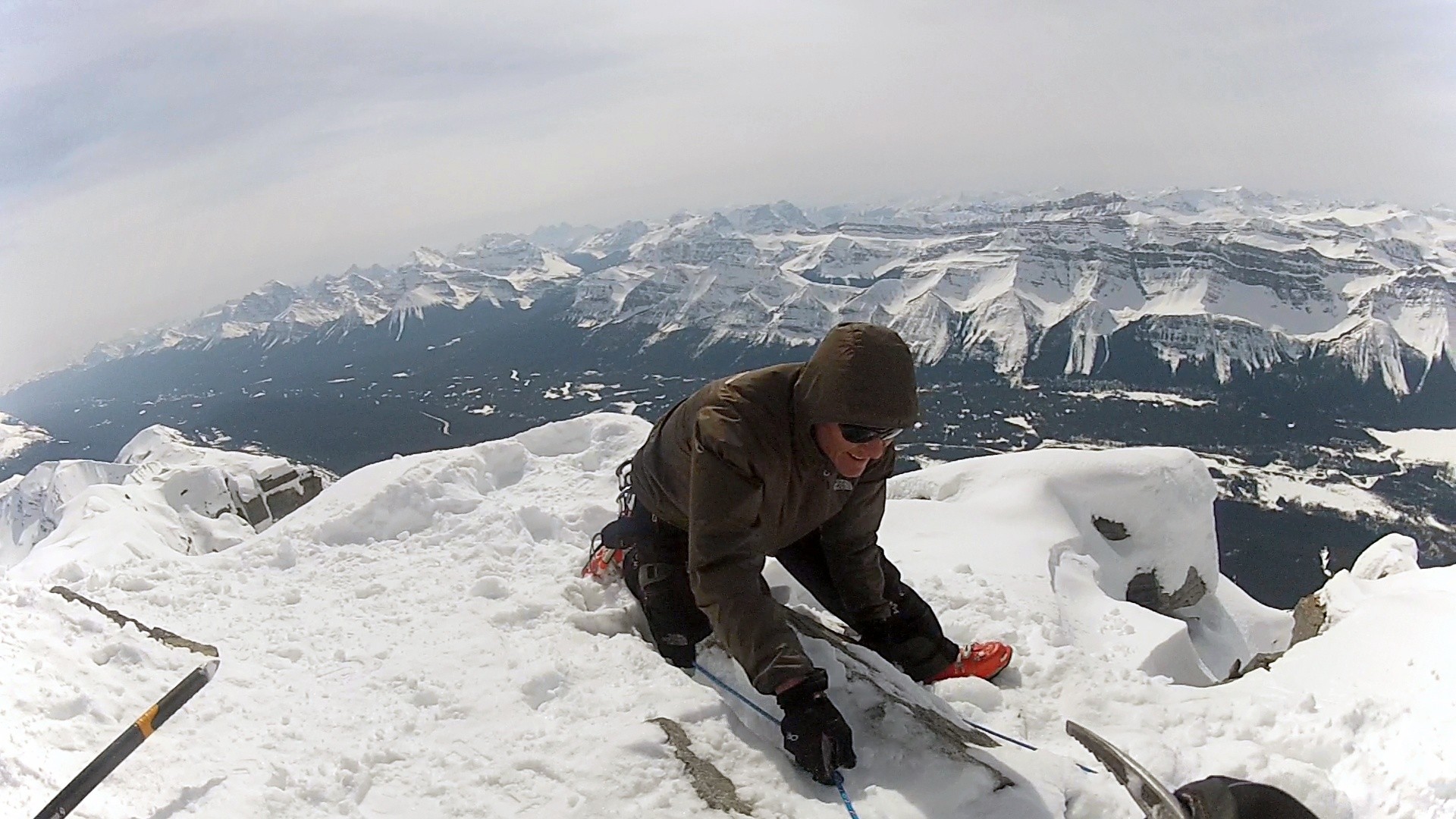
[{"x1": 836, "y1": 424, "x2": 903, "y2": 443}]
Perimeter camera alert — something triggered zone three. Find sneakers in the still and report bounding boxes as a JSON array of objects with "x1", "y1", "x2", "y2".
[
  {"x1": 919, "y1": 640, "x2": 1012, "y2": 683},
  {"x1": 581, "y1": 545, "x2": 628, "y2": 580}
]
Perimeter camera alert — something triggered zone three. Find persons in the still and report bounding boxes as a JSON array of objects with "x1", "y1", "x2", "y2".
[{"x1": 582, "y1": 323, "x2": 1013, "y2": 786}]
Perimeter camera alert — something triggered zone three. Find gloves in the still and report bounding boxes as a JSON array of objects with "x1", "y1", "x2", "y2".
[
  {"x1": 774, "y1": 664, "x2": 858, "y2": 786},
  {"x1": 857, "y1": 613, "x2": 915, "y2": 649}
]
